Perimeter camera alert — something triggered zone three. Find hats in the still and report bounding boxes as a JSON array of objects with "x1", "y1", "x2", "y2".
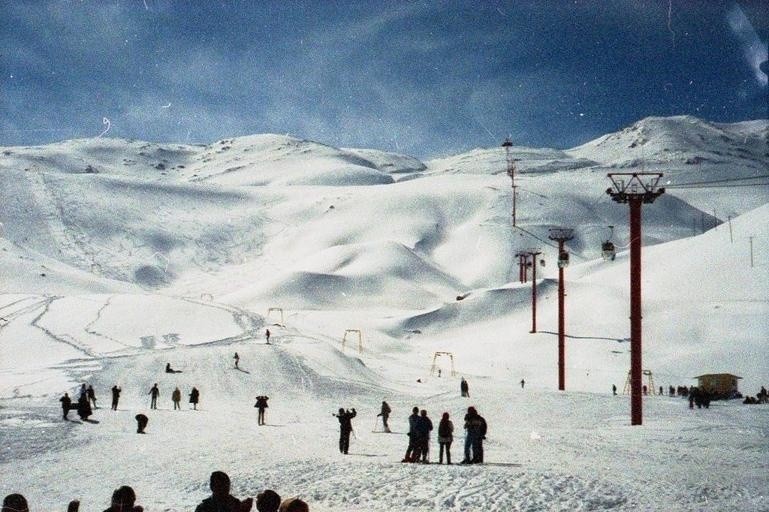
[
  {"x1": 1, "y1": 493, "x2": 29, "y2": 511},
  {"x1": 111, "y1": 484, "x2": 136, "y2": 505},
  {"x1": 209, "y1": 470, "x2": 230, "y2": 491}
]
